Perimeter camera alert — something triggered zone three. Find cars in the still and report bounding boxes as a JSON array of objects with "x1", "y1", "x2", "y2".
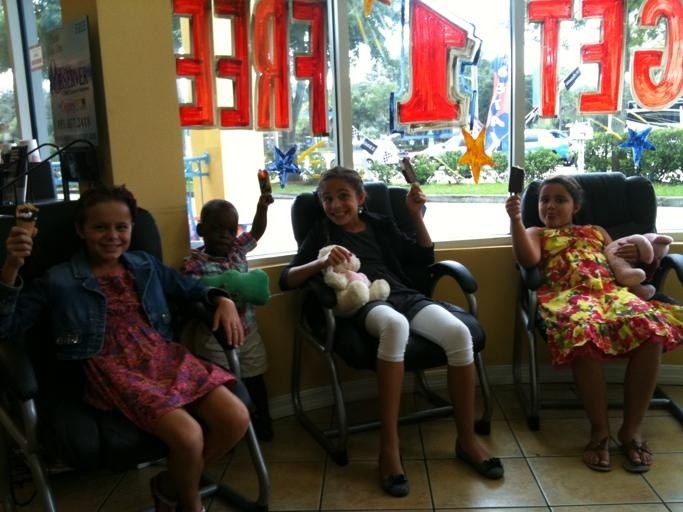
[{"x1": 328, "y1": 97, "x2": 683, "y2": 179}]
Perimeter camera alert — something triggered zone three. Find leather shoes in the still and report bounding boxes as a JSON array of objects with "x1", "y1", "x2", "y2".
[
  {"x1": 455, "y1": 438, "x2": 506, "y2": 481},
  {"x1": 379, "y1": 451, "x2": 409, "y2": 497}
]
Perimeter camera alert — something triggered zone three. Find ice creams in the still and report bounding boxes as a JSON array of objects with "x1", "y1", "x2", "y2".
[{"x1": 16, "y1": 202, "x2": 39, "y2": 238}]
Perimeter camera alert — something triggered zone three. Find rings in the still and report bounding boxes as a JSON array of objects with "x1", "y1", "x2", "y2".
[{"x1": 232, "y1": 326, "x2": 238, "y2": 331}]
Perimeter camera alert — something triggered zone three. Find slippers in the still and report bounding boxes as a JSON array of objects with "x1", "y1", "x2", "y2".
[
  {"x1": 622, "y1": 439, "x2": 653, "y2": 473},
  {"x1": 583, "y1": 437, "x2": 612, "y2": 472}
]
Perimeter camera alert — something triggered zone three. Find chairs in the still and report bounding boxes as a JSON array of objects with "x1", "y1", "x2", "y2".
[
  {"x1": 513, "y1": 173, "x2": 683, "y2": 433},
  {"x1": 288, "y1": 182, "x2": 492, "y2": 465}
]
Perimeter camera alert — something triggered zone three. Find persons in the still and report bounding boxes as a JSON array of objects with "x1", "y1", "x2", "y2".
[
  {"x1": 505, "y1": 175, "x2": 683, "y2": 474},
  {"x1": 278, "y1": 168, "x2": 505, "y2": 497},
  {"x1": 182, "y1": 194, "x2": 274, "y2": 454}
]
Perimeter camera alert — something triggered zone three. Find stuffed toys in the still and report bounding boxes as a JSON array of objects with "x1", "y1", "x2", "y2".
[
  {"x1": 604, "y1": 232, "x2": 673, "y2": 300},
  {"x1": 318, "y1": 244, "x2": 391, "y2": 316}
]
